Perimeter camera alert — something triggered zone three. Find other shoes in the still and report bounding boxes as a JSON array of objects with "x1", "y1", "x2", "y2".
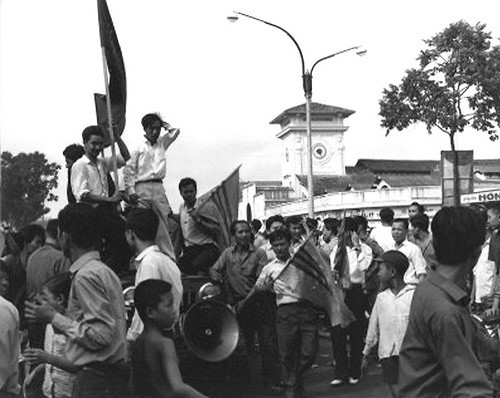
[
  {"x1": 330, "y1": 379, "x2": 343, "y2": 384},
  {"x1": 348, "y1": 376, "x2": 359, "y2": 384}
]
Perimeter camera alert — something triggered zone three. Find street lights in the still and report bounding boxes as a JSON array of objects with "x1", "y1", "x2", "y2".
[{"x1": 227, "y1": 10, "x2": 367, "y2": 218}]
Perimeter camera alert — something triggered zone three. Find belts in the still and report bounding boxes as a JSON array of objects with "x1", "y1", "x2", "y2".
[{"x1": 142, "y1": 178, "x2": 162, "y2": 183}]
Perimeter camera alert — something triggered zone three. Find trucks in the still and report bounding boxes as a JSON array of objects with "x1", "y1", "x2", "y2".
[{"x1": 114, "y1": 271, "x2": 248, "y2": 384}]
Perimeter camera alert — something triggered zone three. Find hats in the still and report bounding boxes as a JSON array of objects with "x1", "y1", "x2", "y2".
[{"x1": 373, "y1": 250, "x2": 408, "y2": 271}]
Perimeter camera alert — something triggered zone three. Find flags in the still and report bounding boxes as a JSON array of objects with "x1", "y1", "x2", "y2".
[
  {"x1": 93, "y1": 0, "x2": 127, "y2": 149},
  {"x1": 278, "y1": 231, "x2": 356, "y2": 329},
  {"x1": 332, "y1": 221, "x2": 352, "y2": 298},
  {"x1": 200, "y1": 165, "x2": 241, "y2": 251}
]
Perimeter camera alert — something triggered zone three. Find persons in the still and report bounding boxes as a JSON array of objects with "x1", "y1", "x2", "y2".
[
  {"x1": 63, "y1": 143, "x2": 116, "y2": 207},
  {"x1": 21, "y1": 270, "x2": 76, "y2": 398},
  {"x1": 178, "y1": 174, "x2": 222, "y2": 281},
  {"x1": 361, "y1": 250, "x2": 418, "y2": 397},
  {"x1": 129, "y1": 279, "x2": 209, "y2": 398},
  {"x1": 328, "y1": 217, "x2": 374, "y2": 385},
  {"x1": 208, "y1": 220, "x2": 285, "y2": 397},
  {"x1": 20, "y1": 202, "x2": 131, "y2": 398},
  {"x1": 253, "y1": 227, "x2": 324, "y2": 398},
  {"x1": 126, "y1": 208, "x2": 183, "y2": 360},
  {"x1": 0, "y1": 295, "x2": 20, "y2": 398},
  {"x1": 397, "y1": 203, "x2": 499, "y2": 398},
  {"x1": 70, "y1": 125, "x2": 130, "y2": 209},
  {"x1": 123, "y1": 112, "x2": 184, "y2": 264},
  {"x1": 0, "y1": 200, "x2": 500, "y2": 325}
]
